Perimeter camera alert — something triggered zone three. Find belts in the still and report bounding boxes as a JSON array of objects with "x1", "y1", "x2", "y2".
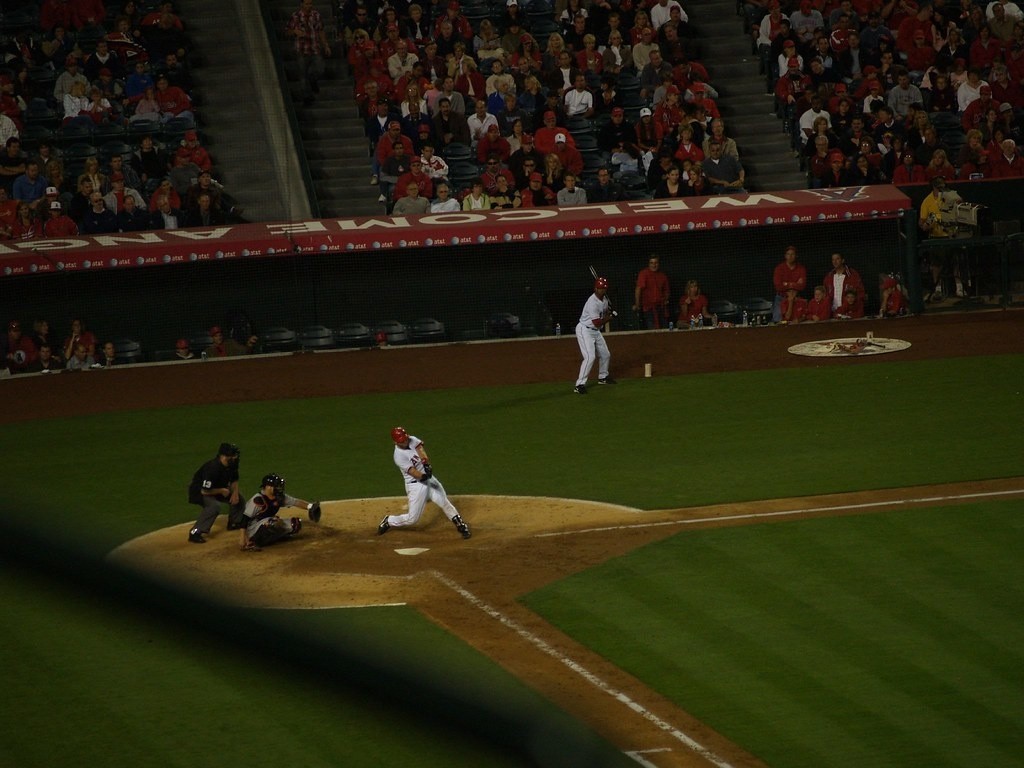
[{"x1": 586, "y1": 326, "x2": 598, "y2": 331}]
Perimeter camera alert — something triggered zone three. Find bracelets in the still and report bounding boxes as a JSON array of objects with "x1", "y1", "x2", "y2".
[{"x1": 740, "y1": 178, "x2": 744, "y2": 181}]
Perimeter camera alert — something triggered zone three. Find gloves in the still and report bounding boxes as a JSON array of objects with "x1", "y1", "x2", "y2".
[
  {"x1": 418, "y1": 474, "x2": 431, "y2": 482},
  {"x1": 424, "y1": 464, "x2": 432, "y2": 473}
]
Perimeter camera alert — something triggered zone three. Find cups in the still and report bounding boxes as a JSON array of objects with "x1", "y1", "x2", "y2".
[
  {"x1": 644, "y1": 363, "x2": 652, "y2": 377},
  {"x1": 669, "y1": 322, "x2": 673, "y2": 332},
  {"x1": 201, "y1": 352, "x2": 207, "y2": 361},
  {"x1": 105, "y1": 360, "x2": 111, "y2": 368}
]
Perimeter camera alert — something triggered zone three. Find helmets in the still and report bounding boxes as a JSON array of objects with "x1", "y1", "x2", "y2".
[
  {"x1": 260, "y1": 474, "x2": 279, "y2": 488},
  {"x1": 594, "y1": 278, "x2": 608, "y2": 289},
  {"x1": 391, "y1": 427, "x2": 408, "y2": 442},
  {"x1": 219, "y1": 443, "x2": 235, "y2": 456}
]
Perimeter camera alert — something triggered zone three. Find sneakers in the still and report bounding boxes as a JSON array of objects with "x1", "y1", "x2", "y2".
[
  {"x1": 378, "y1": 515, "x2": 390, "y2": 535},
  {"x1": 189, "y1": 531, "x2": 205, "y2": 543},
  {"x1": 598, "y1": 377, "x2": 616, "y2": 385},
  {"x1": 243, "y1": 541, "x2": 262, "y2": 552},
  {"x1": 573, "y1": 385, "x2": 587, "y2": 394},
  {"x1": 452, "y1": 515, "x2": 471, "y2": 539},
  {"x1": 226, "y1": 522, "x2": 244, "y2": 530}
]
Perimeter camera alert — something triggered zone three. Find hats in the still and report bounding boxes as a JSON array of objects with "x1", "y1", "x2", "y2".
[
  {"x1": 341, "y1": 0, "x2": 726, "y2": 166},
  {"x1": 209, "y1": 326, "x2": 222, "y2": 336},
  {"x1": 784, "y1": 246, "x2": 797, "y2": 252},
  {"x1": 879, "y1": 279, "x2": 896, "y2": 289},
  {"x1": 767, "y1": 0, "x2": 1014, "y2": 163},
  {"x1": 45, "y1": 67, "x2": 197, "y2": 211},
  {"x1": 786, "y1": 282, "x2": 798, "y2": 291},
  {"x1": 177, "y1": 339, "x2": 188, "y2": 348},
  {"x1": 9, "y1": 320, "x2": 21, "y2": 331}
]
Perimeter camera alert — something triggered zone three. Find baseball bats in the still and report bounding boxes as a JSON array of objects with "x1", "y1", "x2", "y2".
[
  {"x1": 589, "y1": 265, "x2": 614, "y2": 313},
  {"x1": 425, "y1": 477, "x2": 432, "y2": 503}
]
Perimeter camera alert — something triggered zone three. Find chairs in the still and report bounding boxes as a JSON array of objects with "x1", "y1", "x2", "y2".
[
  {"x1": 429, "y1": 0, "x2": 655, "y2": 202},
  {"x1": 740, "y1": 297, "x2": 774, "y2": 324},
  {"x1": 0, "y1": 0, "x2": 220, "y2": 199},
  {"x1": 736, "y1": 0, "x2": 835, "y2": 188},
  {"x1": 707, "y1": 300, "x2": 736, "y2": 326},
  {"x1": 99, "y1": 314, "x2": 521, "y2": 365}
]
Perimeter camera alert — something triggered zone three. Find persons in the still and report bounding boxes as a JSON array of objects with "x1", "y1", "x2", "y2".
[
  {"x1": 287, "y1": 0, "x2": 331, "y2": 108},
  {"x1": 187, "y1": 443, "x2": 244, "y2": 543},
  {"x1": 919, "y1": 175, "x2": 969, "y2": 299},
  {"x1": 376, "y1": 331, "x2": 388, "y2": 347},
  {"x1": 202, "y1": 326, "x2": 259, "y2": 357},
  {"x1": 880, "y1": 277, "x2": 910, "y2": 316},
  {"x1": 770, "y1": 246, "x2": 865, "y2": 321},
  {"x1": 0, "y1": 317, "x2": 118, "y2": 374},
  {"x1": 240, "y1": 474, "x2": 321, "y2": 551},
  {"x1": 677, "y1": 280, "x2": 713, "y2": 327},
  {"x1": 737, "y1": 0, "x2": 1024, "y2": 185},
  {"x1": 574, "y1": 277, "x2": 618, "y2": 394},
  {"x1": 174, "y1": 338, "x2": 199, "y2": 359},
  {"x1": 331, "y1": 0, "x2": 746, "y2": 213},
  {"x1": 632, "y1": 255, "x2": 668, "y2": 328},
  {"x1": 378, "y1": 427, "x2": 471, "y2": 540},
  {"x1": 0, "y1": 0, "x2": 241, "y2": 240}
]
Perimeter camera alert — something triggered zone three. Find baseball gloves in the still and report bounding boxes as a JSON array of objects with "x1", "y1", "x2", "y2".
[{"x1": 308, "y1": 502, "x2": 321, "y2": 523}]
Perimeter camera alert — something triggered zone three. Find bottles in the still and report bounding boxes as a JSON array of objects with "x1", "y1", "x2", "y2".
[
  {"x1": 691, "y1": 315, "x2": 695, "y2": 330},
  {"x1": 743, "y1": 311, "x2": 748, "y2": 327},
  {"x1": 698, "y1": 314, "x2": 703, "y2": 329},
  {"x1": 752, "y1": 313, "x2": 766, "y2": 328},
  {"x1": 712, "y1": 313, "x2": 717, "y2": 327},
  {"x1": 556, "y1": 323, "x2": 561, "y2": 339}
]
